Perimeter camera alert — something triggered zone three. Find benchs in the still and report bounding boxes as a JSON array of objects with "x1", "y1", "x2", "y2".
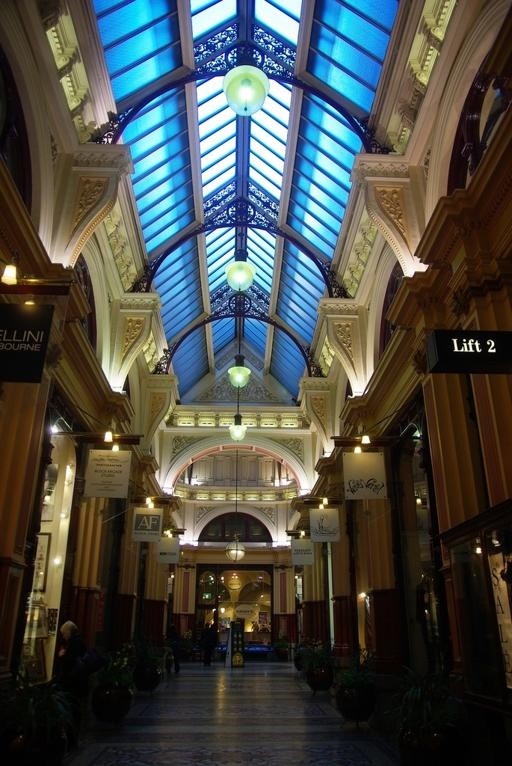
[
  {"x1": 83, "y1": 449, "x2": 132, "y2": 500},
  {"x1": 342, "y1": 451, "x2": 388, "y2": 501},
  {"x1": 132, "y1": 507, "x2": 164, "y2": 542},
  {"x1": 157, "y1": 537, "x2": 180, "y2": 564},
  {"x1": 309, "y1": 508, "x2": 340, "y2": 544},
  {"x1": 290, "y1": 539, "x2": 314, "y2": 565}
]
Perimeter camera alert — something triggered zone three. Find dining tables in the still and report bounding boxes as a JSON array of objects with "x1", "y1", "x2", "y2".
[
  {"x1": 33, "y1": 648, "x2": 162, "y2": 766},
  {"x1": 293, "y1": 642, "x2": 463, "y2": 766}
]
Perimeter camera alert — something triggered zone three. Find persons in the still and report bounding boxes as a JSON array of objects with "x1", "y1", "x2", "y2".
[
  {"x1": 163, "y1": 624, "x2": 181, "y2": 674},
  {"x1": 200, "y1": 622, "x2": 214, "y2": 667},
  {"x1": 52, "y1": 618, "x2": 86, "y2": 750}
]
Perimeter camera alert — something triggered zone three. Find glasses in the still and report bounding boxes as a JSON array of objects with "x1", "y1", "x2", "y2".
[
  {"x1": 290, "y1": 435, "x2": 374, "y2": 539},
  {"x1": 226, "y1": 571, "x2": 243, "y2": 592},
  {"x1": 0, "y1": 264, "x2": 18, "y2": 286},
  {"x1": 103, "y1": 431, "x2": 173, "y2": 538},
  {"x1": 222, "y1": 38, "x2": 270, "y2": 563}
]
